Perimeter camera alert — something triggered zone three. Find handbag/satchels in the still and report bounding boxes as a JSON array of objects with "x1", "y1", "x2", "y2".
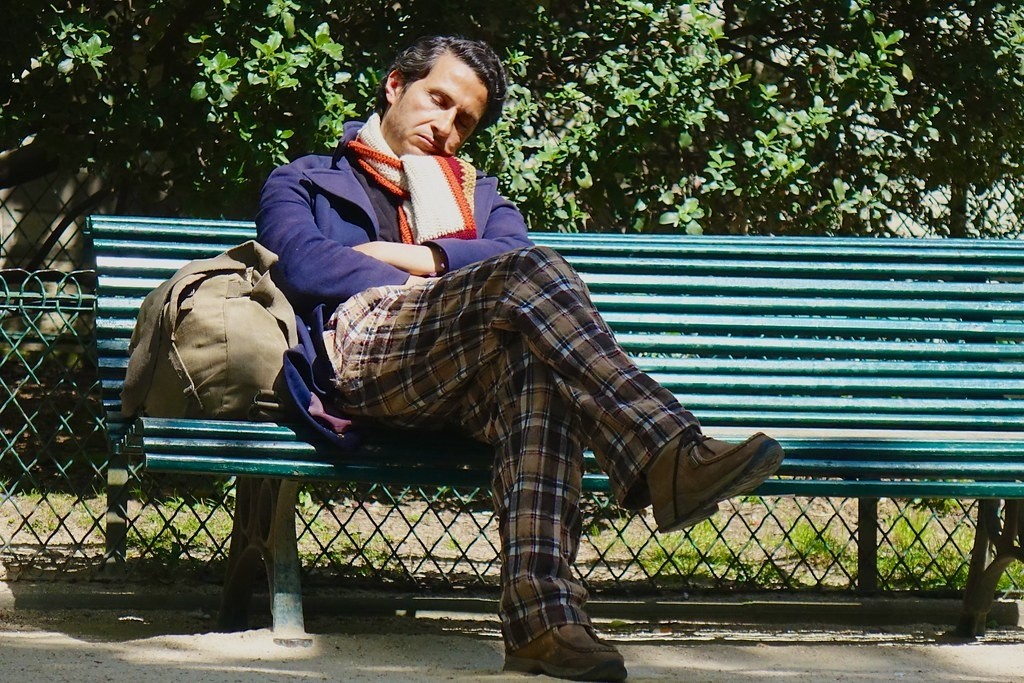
[{"x1": 120, "y1": 239, "x2": 298, "y2": 423}]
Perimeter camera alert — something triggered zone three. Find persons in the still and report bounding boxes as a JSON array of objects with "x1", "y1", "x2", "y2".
[{"x1": 257, "y1": 35, "x2": 784, "y2": 679}]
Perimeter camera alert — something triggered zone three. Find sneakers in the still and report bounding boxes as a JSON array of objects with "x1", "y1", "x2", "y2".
[
  {"x1": 502, "y1": 624, "x2": 627, "y2": 682},
  {"x1": 650, "y1": 424, "x2": 785, "y2": 534}
]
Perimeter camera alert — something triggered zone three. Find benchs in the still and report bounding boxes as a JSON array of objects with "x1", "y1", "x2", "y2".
[{"x1": 85, "y1": 215, "x2": 1024, "y2": 647}]
